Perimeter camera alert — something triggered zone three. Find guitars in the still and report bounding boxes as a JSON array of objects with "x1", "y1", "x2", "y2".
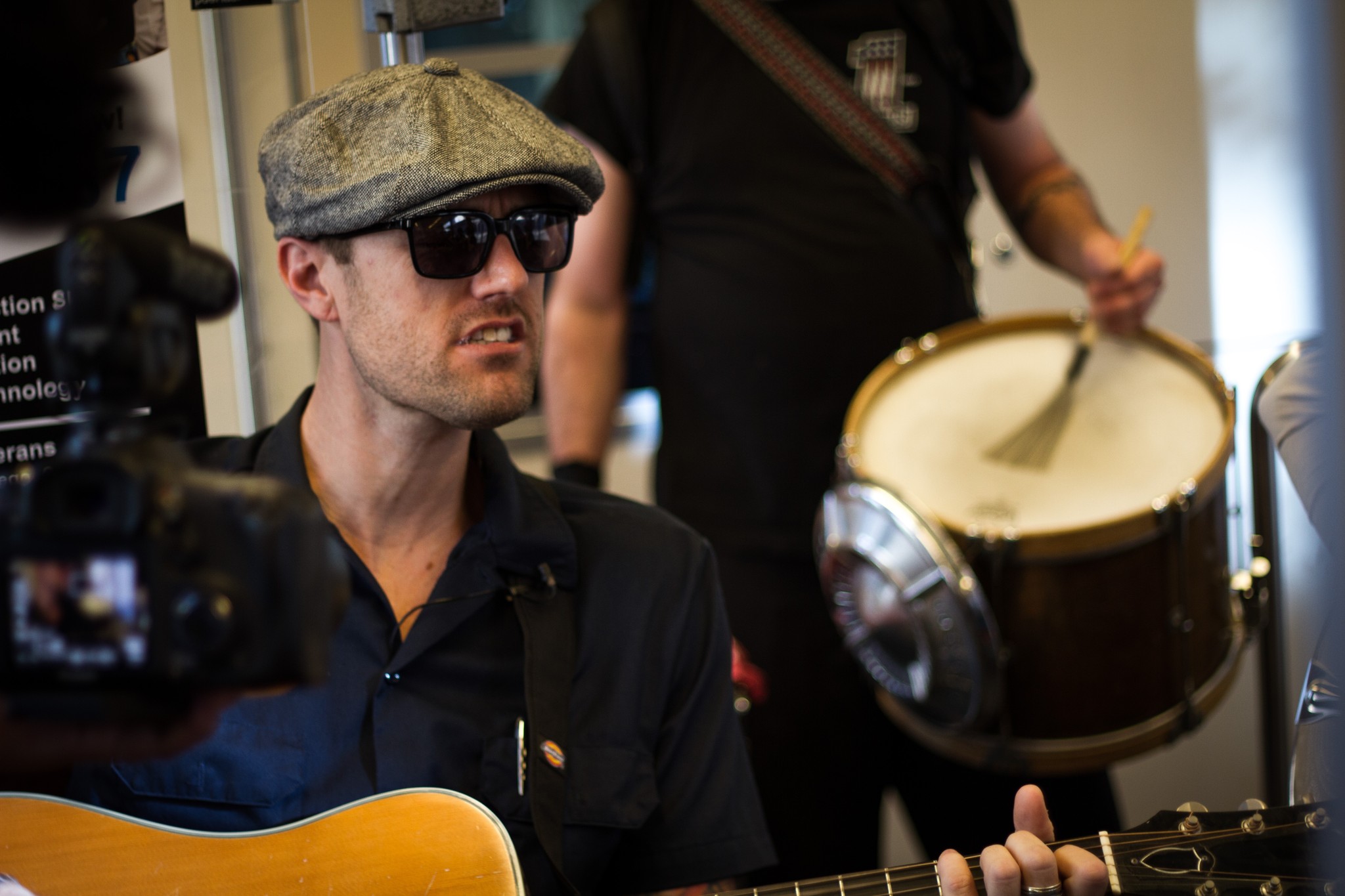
[{"x1": 0, "y1": 781, "x2": 1343, "y2": 896}]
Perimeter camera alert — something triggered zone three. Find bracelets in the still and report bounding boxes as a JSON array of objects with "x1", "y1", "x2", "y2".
[{"x1": 548, "y1": 458, "x2": 600, "y2": 489}]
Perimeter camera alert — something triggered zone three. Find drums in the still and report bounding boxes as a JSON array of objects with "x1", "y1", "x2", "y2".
[{"x1": 813, "y1": 310, "x2": 1248, "y2": 773}]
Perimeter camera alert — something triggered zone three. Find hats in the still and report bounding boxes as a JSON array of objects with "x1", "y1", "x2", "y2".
[{"x1": 256, "y1": 58, "x2": 605, "y2": 240}]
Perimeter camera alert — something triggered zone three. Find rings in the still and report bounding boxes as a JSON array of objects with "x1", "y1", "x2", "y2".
[{"x1": 1022, "y1": 881, "x2": 1063, "y2": 896}]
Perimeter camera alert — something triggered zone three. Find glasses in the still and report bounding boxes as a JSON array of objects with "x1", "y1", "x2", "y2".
[{"x1": 332, "y1": 198, "x2": 579, "y2": 279}]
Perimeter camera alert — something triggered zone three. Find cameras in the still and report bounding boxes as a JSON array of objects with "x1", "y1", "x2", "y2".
[{"x1": 1, "y1": 216, "x2": 327, "y2": 691}]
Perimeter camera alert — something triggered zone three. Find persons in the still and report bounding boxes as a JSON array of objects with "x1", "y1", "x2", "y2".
[
  {"x1": 9, "y1": 59, "x2": 1108, "y2": 896},
  {"x1": 527, "y1": 1, "x2": 1168, "y2": 896}
]
